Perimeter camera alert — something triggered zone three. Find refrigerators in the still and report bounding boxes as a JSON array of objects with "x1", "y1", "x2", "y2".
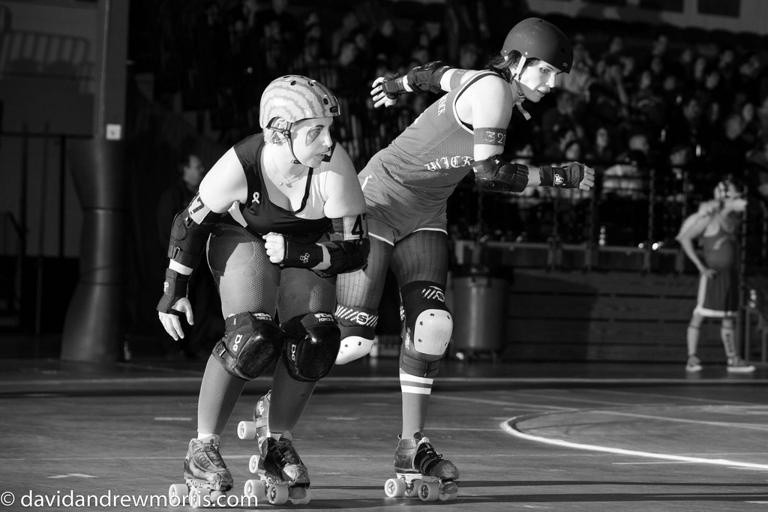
[
  {"x1": 236, "y1": 391, "x2": 311, "y2": 505},
  {"x1": 168, "y1": 434, "x2": 234, "y2": 508},
  {"x1": 384, "y1": 430, "x2": 459, "y2": 501}
]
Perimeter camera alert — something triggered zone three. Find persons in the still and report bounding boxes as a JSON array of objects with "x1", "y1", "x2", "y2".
[
  {"x1": 175, "y1": 1, "x2": 768, "y2": 269},
  {"x1": 238, "y1": 17, "x2": 594, "y2": 500},
  {"x1": 670, "y1": 177, "x2": 759, "y2": 376},
  {"x1": 154, "y1": 73, "x2": 368, "y2": 506},
  {"x1": 155, "y1": 148, "x2": 211, "y2": 369}
]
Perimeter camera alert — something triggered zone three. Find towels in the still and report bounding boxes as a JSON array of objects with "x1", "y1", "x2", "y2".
[{"x1": 453, "y1": 262, "x2": 514, "y2": 361}]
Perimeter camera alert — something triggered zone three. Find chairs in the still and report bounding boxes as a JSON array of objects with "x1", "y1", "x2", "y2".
[
  {"x1": 727, "y1": 361, "x2": 755, "y2": 373},
  {"x1": 686, "y1": 356, "x2": 704, "y2": 373}
]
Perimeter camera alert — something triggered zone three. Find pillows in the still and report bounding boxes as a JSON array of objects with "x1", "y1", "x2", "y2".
[
  {"x1": 259, "y1": 74, "x2": 341, "y2": 130},
  {"x1": 500, "y1": 17, "x2": 574, "y2": 73}
]
